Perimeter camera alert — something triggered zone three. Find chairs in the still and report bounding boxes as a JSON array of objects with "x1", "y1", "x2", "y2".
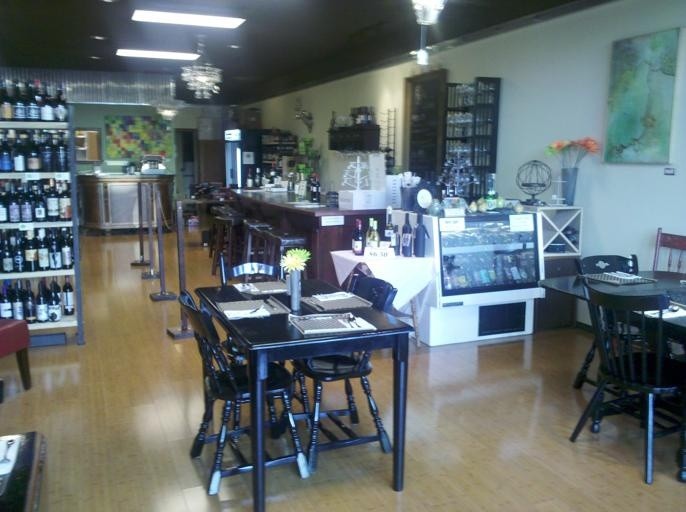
[
  {"x1": 175, "y1": 287, "x2": 311, "y2": 496},
  {"x1": 573, "y1": 249, "x2": 659, "y2": 399},
  {"x1": 568, "y1": 272, "x2": 686, "y2": 487},
  {"x1": 650, "y1": 227, "x2": 686, "y2": 273},
  {"x1": 218, "y1": 251, "x2": 288, "y2": 367},
  {"x1": 273, "y1": 268, "x2": 398, "y2": 474}
]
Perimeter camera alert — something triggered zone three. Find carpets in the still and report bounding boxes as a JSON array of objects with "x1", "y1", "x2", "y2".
[{"x1": 0, "y1": 430, "x2": 47, "y2": 512}]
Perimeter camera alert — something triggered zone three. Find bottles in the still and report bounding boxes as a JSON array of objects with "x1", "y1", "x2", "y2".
[
  {"x1": 286, "y1": 171, "x2": 321, "y2": 203},
  {"x1": 0, "y1": 80, "x2": 74, "y2": 327},
  {"x1": 247, "y1": 167, "x2": 262, "y2": 188},
  {"x1": 353, "y1": 211, "x2": 427, "y2": 257}
]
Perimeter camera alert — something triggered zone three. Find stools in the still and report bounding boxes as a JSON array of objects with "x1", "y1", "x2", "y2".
[
  {"x1": 0, "y1": 317, "x2": 32, "y2": 404},
  {"x1": 206, "y1": 201, "x2": 310, "y2": 282}
]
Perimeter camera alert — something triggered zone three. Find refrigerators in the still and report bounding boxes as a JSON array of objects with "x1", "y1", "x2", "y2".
[{"x1": 224, "y1": 128, "x2": 298, "y2": 190}]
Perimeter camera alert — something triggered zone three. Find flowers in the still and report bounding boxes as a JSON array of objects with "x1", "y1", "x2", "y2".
[{"x1": 545, "y1": 135, "x2": 600, "y2": 169}]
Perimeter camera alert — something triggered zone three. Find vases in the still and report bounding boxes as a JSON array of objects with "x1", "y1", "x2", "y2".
[
  {"x1": 560, "y1": 167, "x2": 578, "y2": 206},
  {"x1": 288, "y1": 271, "x2": 301, "y2": 312}
]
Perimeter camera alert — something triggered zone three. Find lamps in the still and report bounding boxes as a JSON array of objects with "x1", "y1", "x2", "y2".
[
  {"x1": 409, "y1": 0, "x2": 446, "y2": 68},
  {"x1": 179, "y1": 14, "x2": 223, "y2": 100}
]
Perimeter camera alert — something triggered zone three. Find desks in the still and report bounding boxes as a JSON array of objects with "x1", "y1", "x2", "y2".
[
  {"x1": 191, "y1": 278, "x2": 415, "y2": 512},
  {"x1": 535, "y1": 268, "x2": 686, "y2": 484}
]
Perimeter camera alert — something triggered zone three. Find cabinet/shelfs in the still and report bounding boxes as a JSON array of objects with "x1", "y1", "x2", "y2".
[
  {"x1": 329, "y1": 248, "x2": 436, "y2": 350},
  {"x1": 522, "y1": 202, "x2": 584, "y2": 333},
  {"x1": 0, "y1": 94, "x2": 86, "y2": 349},
  {"x1": 74, "y1": 128, "x2": 102, "y2": 163},
  {"x1": 241, "y1": 126, "x2": 298, "y2": 184},
  {"x1": 440, "y1": 75, "x2": 502, "y2": 202},
  {"x1": 198, "y1": 136, "x2": 226, "y2": 230},
  {"x1": 384, "y1": 200, "x2": 548, "y2": 350}
]
